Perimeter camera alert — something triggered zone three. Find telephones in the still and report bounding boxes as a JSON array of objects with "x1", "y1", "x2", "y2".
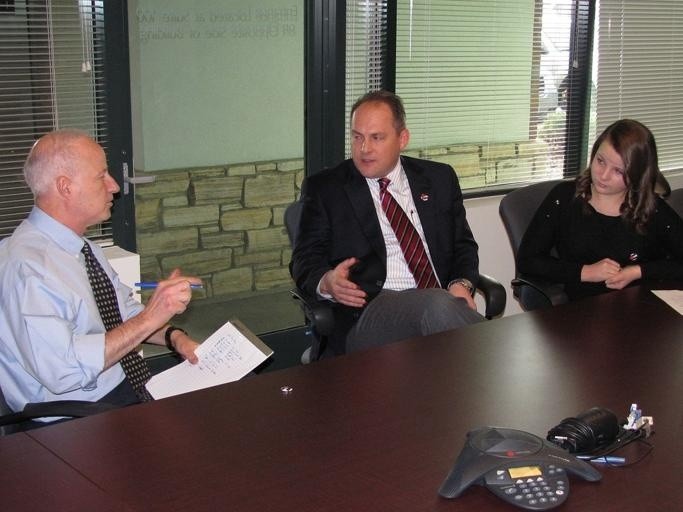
[{"x1": 435, "y1": 426, "x2": 602, "y2": 509}]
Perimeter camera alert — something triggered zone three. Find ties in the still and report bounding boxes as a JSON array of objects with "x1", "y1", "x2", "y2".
[
  {"x1": 81, "y1": 239, "x2": 156, "y2": 403},
  {"x1": 378, "y1": 176, "x2": 441, "y2": 290}
]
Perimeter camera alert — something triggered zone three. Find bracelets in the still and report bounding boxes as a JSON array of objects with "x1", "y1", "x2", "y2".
[{"x1": 164, "y1": 326, "x2": 189, "y2": 361}]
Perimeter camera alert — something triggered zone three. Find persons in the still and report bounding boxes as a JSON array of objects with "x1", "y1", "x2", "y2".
[
  {"x1": 515, "y1": 118, "x2": 681, "y2": 313},
  {"x1": 0, "y1": 127, "x2": 207, "y2": 433},
  {"x1": 287, "y1": 90, "x2": 489, "y2": 366}
]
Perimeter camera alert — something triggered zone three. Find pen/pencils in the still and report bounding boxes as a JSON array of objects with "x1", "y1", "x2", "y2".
[{"x1": 135, "y1": 283, "x2": 203, "y2": 289}]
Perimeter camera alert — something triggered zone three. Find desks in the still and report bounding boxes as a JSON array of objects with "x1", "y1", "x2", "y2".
[{"x1": 2, "y1": 286, "x2": 683, "y2": 512}]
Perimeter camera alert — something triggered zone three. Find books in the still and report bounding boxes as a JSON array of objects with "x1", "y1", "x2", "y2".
[{"x1": 142, "y1": 313, "x2": 275, "y2": 401}]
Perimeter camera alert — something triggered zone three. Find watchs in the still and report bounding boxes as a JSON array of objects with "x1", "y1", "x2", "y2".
[{"x1": 445, "y1": 278, "x2": 475, "y2": 298}]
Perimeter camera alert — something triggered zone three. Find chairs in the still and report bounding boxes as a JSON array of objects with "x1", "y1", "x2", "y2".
[
  {"x1": 282, "y1": 197, "x2": 512, "y2": 363},
  {"x1": 500, "y1": 178, "x2": 578, "y2": 313}
]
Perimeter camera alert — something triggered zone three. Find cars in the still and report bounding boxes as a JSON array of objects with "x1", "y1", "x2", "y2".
[{"x1": 539, "y1": 15, "x2": 571, "y2": 110}]
